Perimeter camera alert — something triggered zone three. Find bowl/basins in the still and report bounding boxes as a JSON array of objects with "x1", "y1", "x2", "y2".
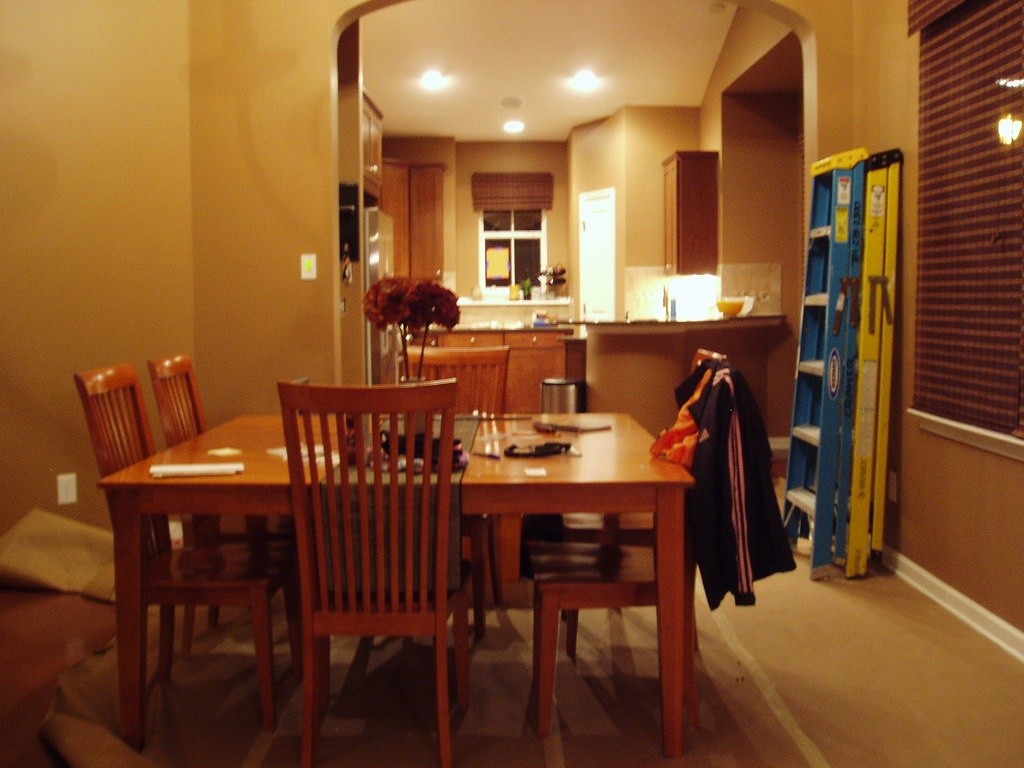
[{"x1": 716, "y1": 296, "x2": 754, "y2": 317}]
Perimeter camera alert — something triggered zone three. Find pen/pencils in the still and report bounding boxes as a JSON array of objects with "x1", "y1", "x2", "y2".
[{"x1": 152, "y1": 471, "x2": 241, "y2": 478}]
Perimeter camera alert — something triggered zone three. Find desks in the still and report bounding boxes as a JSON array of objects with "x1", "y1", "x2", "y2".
[{"x1": 95, "y1": 412, "x2": 696, "y2": 757}]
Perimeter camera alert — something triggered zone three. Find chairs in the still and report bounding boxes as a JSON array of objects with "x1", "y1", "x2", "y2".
[
  {"x1": 146, "y1": 355, "x2": 302, "y2": 658},
  {"x1": 70, "y1": 366, "x2": 306, "y2": 753},
  {"x1": 531, "y1": 348, "x2": 796, "y2": 737},
  {"x1": 360, "y1": 343, "x2": 509, "y2": 648},
  {"x1": 277, "y1": 379, "x2": 468, "y2": 768}
]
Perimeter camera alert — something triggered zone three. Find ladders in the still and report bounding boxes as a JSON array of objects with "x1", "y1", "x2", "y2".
[{"x1": 783, "y1": 146, "x2": 902, "y2": 581}]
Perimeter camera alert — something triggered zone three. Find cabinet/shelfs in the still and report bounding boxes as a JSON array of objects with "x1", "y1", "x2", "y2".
[
  {"x1": 408, "y1": 331, "x2": 567, "y2": 412},
  {"x1": 661, "y1": 150, "x2": 719, "y2": 276}
]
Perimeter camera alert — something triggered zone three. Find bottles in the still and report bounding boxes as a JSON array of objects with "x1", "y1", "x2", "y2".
[{"x1": 521, "y1": 273, "x2": 532, "y2": 300}]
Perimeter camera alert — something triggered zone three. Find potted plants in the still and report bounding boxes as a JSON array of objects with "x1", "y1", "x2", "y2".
[{"x1": 521, "y1": 278, "x2": 532, "y2": 300}]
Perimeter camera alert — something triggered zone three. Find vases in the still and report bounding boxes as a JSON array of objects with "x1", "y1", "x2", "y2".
[
  {"x1": 400, "y1": 375, "x2": 427, "y2": 435},
  {"x1": 539, "y1": 279, "x2": 548, "y2": 299}
]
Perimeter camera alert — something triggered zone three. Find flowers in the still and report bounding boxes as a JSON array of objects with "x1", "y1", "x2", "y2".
[
  {"x1": 361, "y1": 277, "x2": 461, "y2": 378},
  {"x1": 538, "y1": 261, "x2": 566, "y2": 286}
]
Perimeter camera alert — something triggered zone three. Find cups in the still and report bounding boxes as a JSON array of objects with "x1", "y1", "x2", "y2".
[{"x1": 508, "y1": 284, "x2": 521, "y2": 300}]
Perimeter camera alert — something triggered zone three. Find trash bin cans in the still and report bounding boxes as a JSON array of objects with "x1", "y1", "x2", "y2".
[{"x1": 542, "y1": 376, "x2": 586, "y2": 413}]
